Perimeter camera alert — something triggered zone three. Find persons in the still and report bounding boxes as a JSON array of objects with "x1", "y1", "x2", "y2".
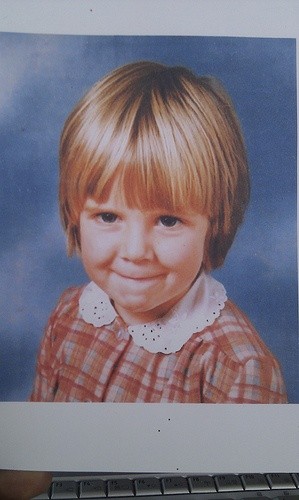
[{"x1": 26, "y1": 60, "x2": 285, "y2": 403}]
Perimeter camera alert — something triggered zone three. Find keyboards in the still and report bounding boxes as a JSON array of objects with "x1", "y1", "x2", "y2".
[{"x1": 48, "y1": 471, "x2": 299, "y2": 500}]
